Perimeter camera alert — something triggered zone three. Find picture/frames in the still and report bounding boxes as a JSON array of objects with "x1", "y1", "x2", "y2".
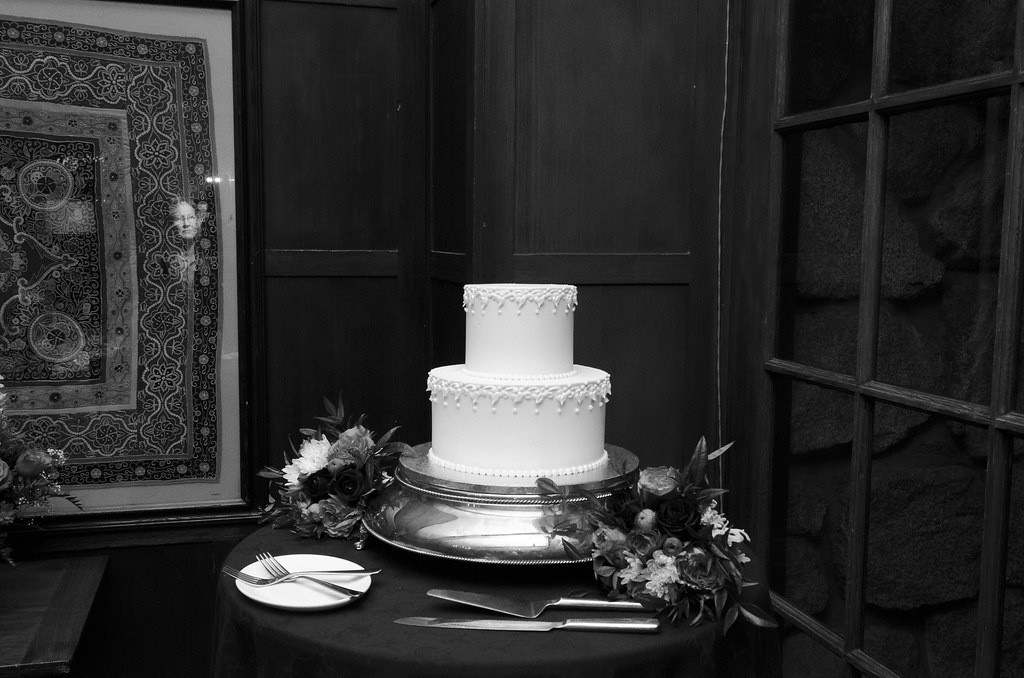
[{"x1": 0, "y1": 0, "x2": 276, "y2": 535}]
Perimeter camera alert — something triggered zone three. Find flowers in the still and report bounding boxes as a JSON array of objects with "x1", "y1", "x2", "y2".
[
  {"x1": 536, "y1": 435, "x2": 777, "y2": 633},
  {"x1": 254, "y1": 391, "x2": 418, "y2": 552},
  {"x1": 0, "y1": 374, "x2": 86, "y2": 567}
]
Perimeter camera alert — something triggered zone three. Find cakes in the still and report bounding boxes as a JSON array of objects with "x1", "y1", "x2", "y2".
[{"x1": 427, "y1": 283, "x2": 612, "y2": 476}]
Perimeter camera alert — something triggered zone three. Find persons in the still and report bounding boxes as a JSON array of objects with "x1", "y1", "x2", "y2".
[{"x1": 164, "y1": 195, "x2": 204, "y2": 287}]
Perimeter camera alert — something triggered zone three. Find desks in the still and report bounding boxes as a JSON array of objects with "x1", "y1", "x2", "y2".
[
  {"x1": 213, "y1": 526, "x2": 729, "y2": 677},
  {"x1": 0, "y1": 554, "x2": 114, "y2": 678}
]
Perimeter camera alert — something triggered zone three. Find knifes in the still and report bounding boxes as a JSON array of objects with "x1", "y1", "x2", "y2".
[
  {"x1": 426, "y1": 588, "x2": 651, "y2": 619},
  {"x1": 394, "y1": 617, "x2": 660, "y2": 632}
]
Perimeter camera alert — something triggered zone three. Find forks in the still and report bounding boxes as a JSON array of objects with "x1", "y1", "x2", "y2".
[
  {"x1": 255, "y1": 552, "x2": 362, "y2": 598},
  {"x1": 221, "y1": 564, "x2": 383, "y2": 586}
]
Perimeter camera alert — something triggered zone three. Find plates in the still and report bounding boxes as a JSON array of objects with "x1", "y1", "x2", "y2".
[{"x1": 236, "y1": 553, "x2": 372, "y2": 611}]
